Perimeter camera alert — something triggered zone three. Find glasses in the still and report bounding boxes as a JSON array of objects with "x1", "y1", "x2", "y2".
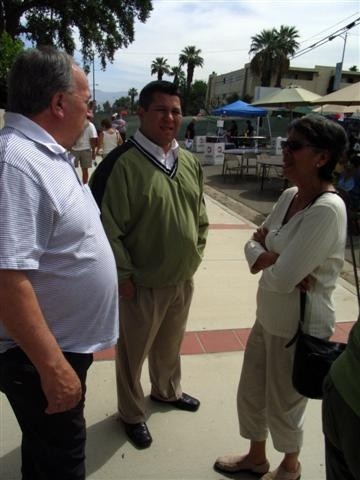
[
  {"x1": 280, "y1": 141, "x2": 322, "y2": 151},
  {"x1": 46, "y1": 87, "x2": 97, "y2": 113}
]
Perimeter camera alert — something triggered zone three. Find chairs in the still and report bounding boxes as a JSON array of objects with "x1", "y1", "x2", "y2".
[{"x1": 222, "y1": 151, "x2": 260, "y2": 179}]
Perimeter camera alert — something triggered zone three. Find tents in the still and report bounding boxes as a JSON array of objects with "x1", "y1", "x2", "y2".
[{"x1": 212, "y1": 99, "x2": 270, "y2": 148}]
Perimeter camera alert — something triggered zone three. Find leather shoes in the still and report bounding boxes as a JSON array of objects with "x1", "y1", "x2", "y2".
[
  {"x1": 118, "y1": 415, "x2": 152, "y2": 448},
  {"x1": 150, "y1": 392, "x2": 200, "y2": 411}
]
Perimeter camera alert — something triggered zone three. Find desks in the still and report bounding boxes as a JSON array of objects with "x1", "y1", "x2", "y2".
[
  {"x1": 222, "y1": 149, "x2": 262, "y2": 178},
  {"x1": 257, "y1": 161, "x2": 289, "y2": 192}
]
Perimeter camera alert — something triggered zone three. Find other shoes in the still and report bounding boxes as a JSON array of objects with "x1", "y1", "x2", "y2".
[
  {"x1": 261, "y1": 463, "x2": 302, "y2": 480},
  {"x1": 213, "y1": 456, "x2": 270, "y2": 480}
]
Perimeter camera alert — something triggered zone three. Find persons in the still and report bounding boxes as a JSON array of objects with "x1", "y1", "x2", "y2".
[
  {"x1": 185, "y1": 117, "x2": 198, "y2": 151},
  {"x1": 213, "y1": 116, "x2": 347, "y2": 480},
  {"x1": 321, "y1": 317, "x2": 360, "y2": 479},
  {"x1": 92, "y1": 80, "x2": 210, "y2": 451},
  {"x1": 218, "y1": 119, "x2": 254, "y2": 142},
  {"x1": 0, "y1": 49, "x2": 120, "y2": 480},
  {"x1": 71, "y1": 113, "x2": 128, "y2": 185}
]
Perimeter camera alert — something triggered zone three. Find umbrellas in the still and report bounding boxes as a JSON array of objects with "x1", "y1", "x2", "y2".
[{"x1": 249, "y1": 81, "x2": 360, "y2": 135}]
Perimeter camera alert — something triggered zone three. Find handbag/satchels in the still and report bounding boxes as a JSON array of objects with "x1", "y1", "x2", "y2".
[{"x1": 285, "y1": 330, "x2": 348, "y2": 400}]
